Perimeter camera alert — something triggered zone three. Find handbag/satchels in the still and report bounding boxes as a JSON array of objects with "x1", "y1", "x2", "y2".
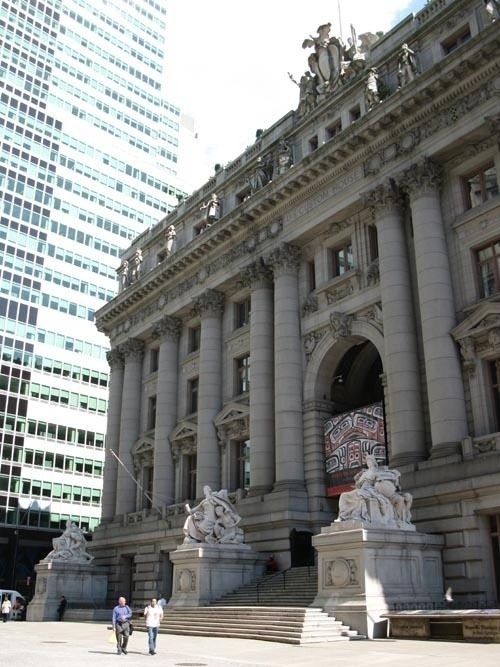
[{"x1": 128, "y1": 624, "x2": 133, "y2": 635}]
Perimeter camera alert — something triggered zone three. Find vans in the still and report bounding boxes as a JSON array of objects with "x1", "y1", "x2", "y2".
[{"x1": 0, "y1": 589, "x2": 25, "y2": 615}]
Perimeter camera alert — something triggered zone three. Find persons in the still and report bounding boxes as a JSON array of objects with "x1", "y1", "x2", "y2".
[
  {"x1": 12, "y1": 597, "x2": 21, "y2": 621},
  {"x1": 1, "y1": 595, "x2": 12, "y2": 624},
  {"x1": 45, "y1": 519, "x2": 97, "y2": 564},
  {"x1": 114, "y1": 138, "x2": 291, "y2": 290},
  {"x1": 333, "y1": 452, "x2": 414, "y2": 526},
  {"x1": 143, "y1": 597, "x2": 166, "y2": 655},
  {"x1": 300, "y1": 70, "x2": 314, "y2": 104},
  {"x1": 56, "y1": 595, "x2": 68, "y2": 621},
  {"x1": 158, "y1": 593, "x2": 168, "y2": 605},
  {"x1": 112, "y1": 595, "x2": 135, "y2": 654},
  {"x1": 364, "y1": 64, "x2": 384, "y2": 110},
  {"x1": 182, "y1": 483, "x2": 245, "y2": 546},
  {"x1": 396, "y1": 42, "x2": 415, "y2": 92}
]
